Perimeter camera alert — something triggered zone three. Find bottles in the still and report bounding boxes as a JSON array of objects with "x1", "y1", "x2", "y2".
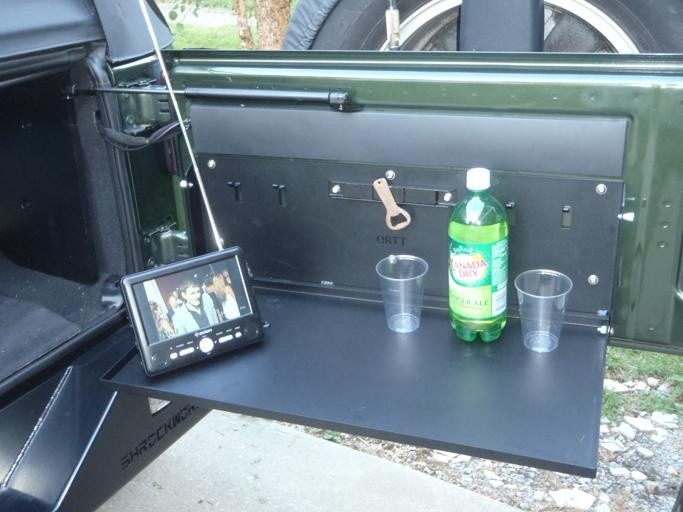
[{"x1": 445, "y1": 166, "x2": 510, "y2": 344}]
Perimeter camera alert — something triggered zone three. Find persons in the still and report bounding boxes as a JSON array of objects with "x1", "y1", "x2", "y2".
[{"x1": 146, "y1": 270, "x2": 243, "y2": 344}]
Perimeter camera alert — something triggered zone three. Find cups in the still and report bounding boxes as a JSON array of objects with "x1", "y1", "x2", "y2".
[
  {"x1": 515, "y1": 269, "x2": 573, "y2": 351},
  {"x1": 376, "y1": 254, "x2": 428, "y2": 332}
]
